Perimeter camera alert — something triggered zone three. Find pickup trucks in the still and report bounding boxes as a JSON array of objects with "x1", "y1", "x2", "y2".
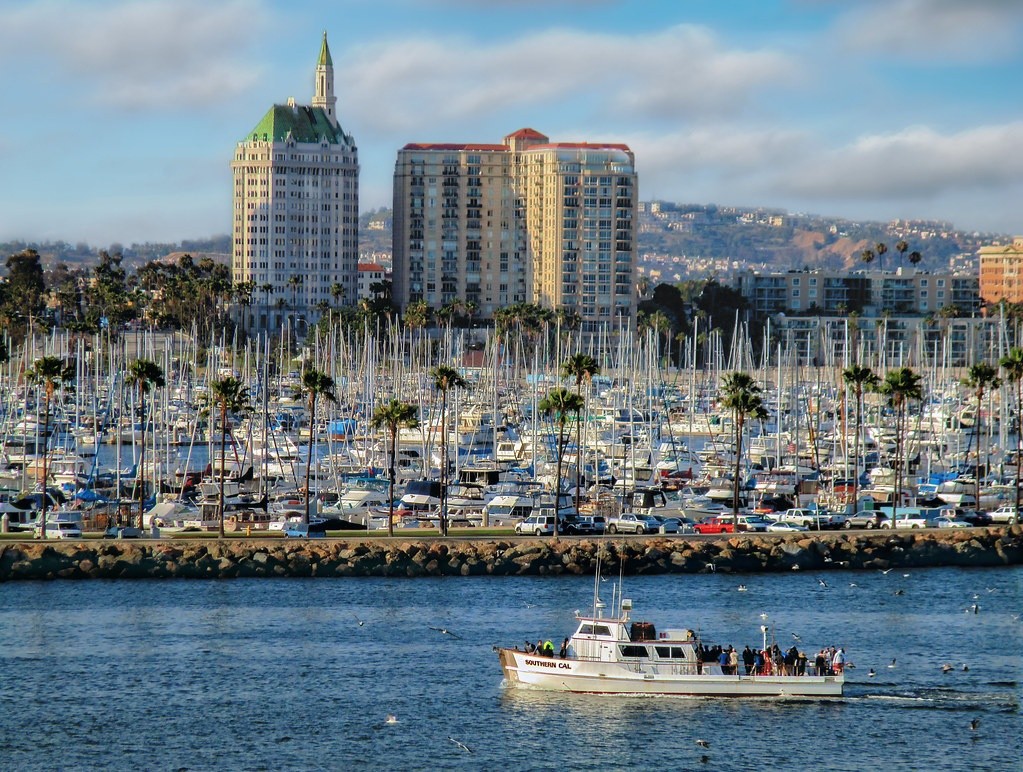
[
  {"x1": 809, "y1": 509, "x2": 847, "y2": 530},
  {"x1": 762, "y1": 508, "x2": 829, "y2": 530},
  {"x1": 604, "y1": 513, "x2": 660, "y2": 534},
  {"x1": 878, "y1": 513, "x2": 932, "y2": 528},
  {"x1": 692, "y1": 518, "x2": 747, "y2": 533}
]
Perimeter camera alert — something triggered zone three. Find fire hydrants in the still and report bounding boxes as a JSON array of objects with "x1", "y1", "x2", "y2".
[{"x1": 246, "y1": 524, "x2": 251, "y2": 537}]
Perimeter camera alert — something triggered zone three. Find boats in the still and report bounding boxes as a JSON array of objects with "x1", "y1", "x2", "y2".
[{"x1": 493, "y1": 530, "x2": 850, "y2": 701}]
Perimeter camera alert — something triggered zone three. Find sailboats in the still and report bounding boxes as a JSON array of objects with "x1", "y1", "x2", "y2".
[{"x1": 0, "y1": 304, "x2": 1023, "y2": 528}]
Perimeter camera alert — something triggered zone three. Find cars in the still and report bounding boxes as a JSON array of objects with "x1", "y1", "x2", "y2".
[
  {"x1": 662, "y1": 517, "x2": 694, "y2": 531},
  {"x1": 985, "y1": 507, "x2": 1023, "y2": 523},
  {"x1": 843, "y1": 511, "x2": 887, "y2": 530},
  {"x1": 102, "y1": 526, "x2": 145, "y2": 539},
  {"x1": 976, "y1": 511, "x2": 991, "y2": 526},
  {"x1": 765, "y1": 521, "x2": 807, "y2": 531},
  {"x1": 284, "y1": 524, "x2": 326, "y2": 539},
  {"x1": 654, "y1": 515, "x2": 667, "y2": 524},
  {"x1": 573, "y1": 515, "x2": 606, "y2": 535},
  {"x1": 563, "y1": 519, "x2": 594, "y2": 536},
  {"x1": 933, "y1": 516, "x2": 973, "y2": 528}
]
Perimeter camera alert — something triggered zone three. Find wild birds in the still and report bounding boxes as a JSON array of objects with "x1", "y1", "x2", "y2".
[
  {"x1": 349, "y1": 611, "x2": 367, "y2": 628},
  {"x1": 601, "y1": 575, "x2": 609, "y2": 582},
  {"x1": 447, "y1": 735, "x2": 472, "y2": 754},
  {"x1": 427, "y1": 625, "x2": 463, "y2": 641},
  {"x1": 694, "y1": 739, "x2": 712, "y2": 748},
  {"x1": 704, "y1": 554, "x2": 1023, "y2": 731},
  {"x1": 385, "y1": 715, "x2": 400, "y2": 724},
  {"x1": 523, "y1": 600, "x2": 541, "y2": 609}
]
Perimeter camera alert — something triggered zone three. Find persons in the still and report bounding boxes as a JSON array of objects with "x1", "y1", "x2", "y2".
[
  {"x1": 523, "y1": 639, "x2": 556, "y2": 658},
  {"x1": 560, "y1": 638, "x2": 569, "y2": 658},
  {"x1": 743, "y1": 646, "x2": 810, "y2": 676},
  {"x1": 694, "y1": 643, "x2": 739, "y2": 675},
  {"x1": 816, "y1": 646, "x2": 844, "y2": 678}
]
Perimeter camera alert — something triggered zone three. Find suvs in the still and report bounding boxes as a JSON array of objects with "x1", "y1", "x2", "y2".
[{"x1": 515, "y1": 514, "x2": 564, "y2": 536}]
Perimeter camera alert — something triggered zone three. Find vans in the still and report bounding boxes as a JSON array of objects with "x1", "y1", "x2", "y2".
[
  {"x1": 715, "y1": 514, "x2": 766, "y2": 532},
  {"x1": 33, "y1": 510, "x2": 85, "y2": 538},
  {"x1": 44, "y1": 522, "x2": 82, "y2": 540}
]
